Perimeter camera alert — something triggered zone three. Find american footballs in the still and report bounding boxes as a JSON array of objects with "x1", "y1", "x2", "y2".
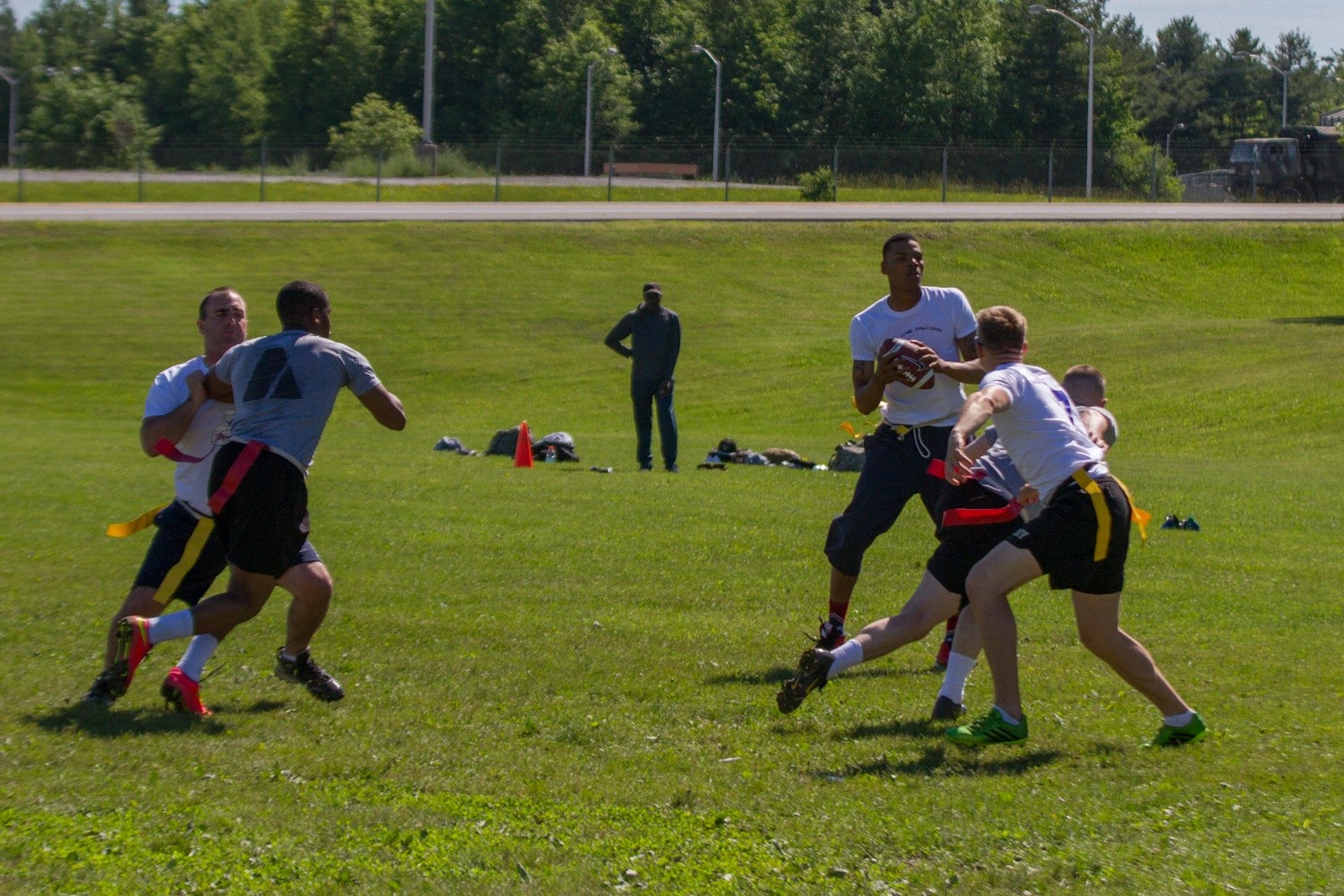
[{"x1": 881, "y1": 338, "x2": 936, "y2": 389}]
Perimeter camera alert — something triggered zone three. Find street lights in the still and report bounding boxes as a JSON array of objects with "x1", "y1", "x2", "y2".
[
  {"x1": 1166, "y1": 122, "x2": 1185, "y2": 156},
  {"x1": 691, "y1": 45, "x2": 721, "y2": 181},
  {"x1": 584, "y1": 47, "x2": 618, "y2": 177},
  {"x1": 1029, "y1": 5, "x2": 1095, "y2": 200},
  {"x1": 1232, "y1": 51, "x2": 1287, "y2": 129}
]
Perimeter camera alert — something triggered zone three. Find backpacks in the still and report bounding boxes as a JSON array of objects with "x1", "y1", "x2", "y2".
[{"x1": 486, "y1": 426, "x2": 534, "y2": 459}]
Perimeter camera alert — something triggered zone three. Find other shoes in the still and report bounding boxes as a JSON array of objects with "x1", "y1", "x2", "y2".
[
  {"x1": 803, "y1": 616, "x2": 845, "y2": 650},
  {"x1": 935, "y1": 642, "x2": 949, "y2": 669}
]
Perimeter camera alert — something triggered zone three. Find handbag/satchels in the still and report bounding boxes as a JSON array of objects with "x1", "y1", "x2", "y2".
[
  {"x1": 829, "y1": 440, "x2": 866, "y2": 472},
  {"x1": 534, "y1": 432, "x2": 579, "y2": 462}
]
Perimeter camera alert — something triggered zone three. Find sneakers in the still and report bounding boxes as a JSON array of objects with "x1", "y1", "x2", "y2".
[
  {"x1": 915, "y1": 695, "x2": 967, "y2": 736},
  {"x1": 162, "y1": 661, "x2": 228, "y2": 717},
  {"x1": 945, "y1": 708, "x2": 1028, "y2": 749},
  {"x1": 1144, "y1": 714, "x2": 1209, "y2": 751},
  {"x1": 82, "y1": 671, "x2": 114, "y2": 708},
  {"x1": 276, "y1": 645, "x2": 344, "y2": 701},
  {"x1": 775, "y1": 648, "x2": 834, "y2": 714},
  {"x1": 109, "y1": 615, "x2": 154, "y2": 697}
]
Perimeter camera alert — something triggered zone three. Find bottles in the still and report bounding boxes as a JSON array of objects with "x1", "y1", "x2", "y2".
[{"x1": 545, "y1": 446, "x2": 556, "y2": 469}]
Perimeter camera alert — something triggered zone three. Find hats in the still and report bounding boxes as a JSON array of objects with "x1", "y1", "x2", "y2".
[{"x1": 643, "y1": 283, "x2": 661, "y2": 296}]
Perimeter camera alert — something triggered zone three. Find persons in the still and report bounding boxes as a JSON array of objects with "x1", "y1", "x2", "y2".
[
  {"x1": 776, "y1": 233, "x2": 1209, "y2": 750},
  {"x1": 603, "y1": 283, "x2": 681, "y2": 473},
  {"x1": 79, "y1": 280, "x2": 406, "y2": 719}
]
